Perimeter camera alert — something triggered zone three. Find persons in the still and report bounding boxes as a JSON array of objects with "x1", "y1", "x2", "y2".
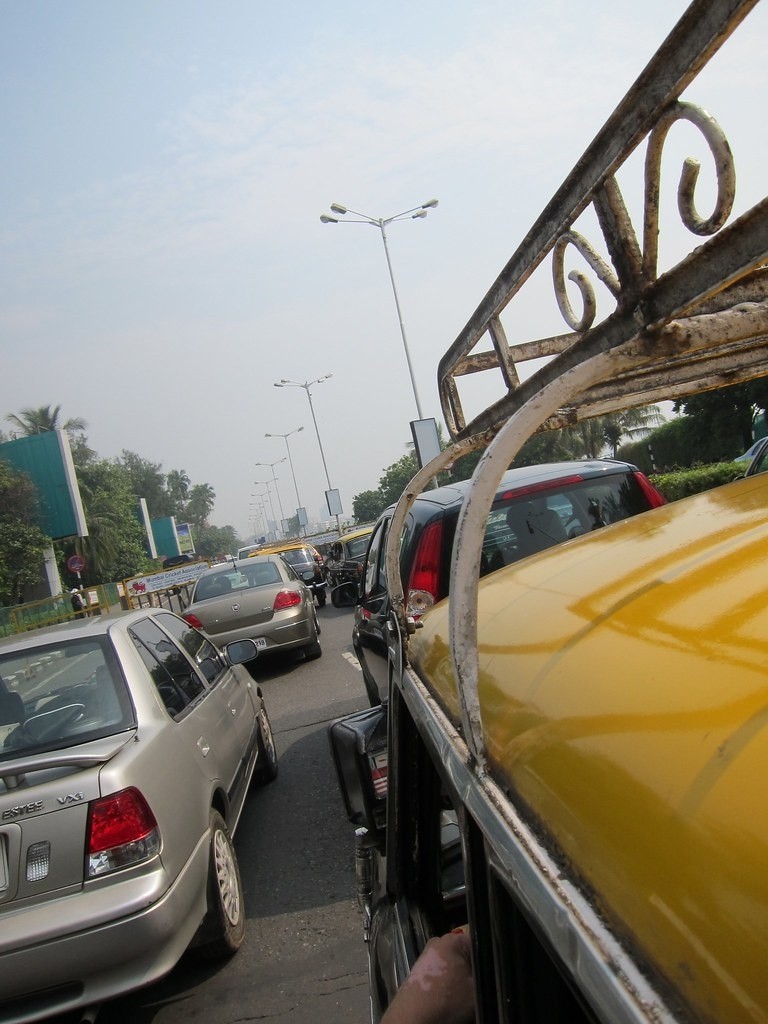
[
  {"x1": 504, "y1": 502, "x2": 558, "y2": 560},
  {"x1": 379, "y1": 931, "x2": 477, "y2": 1024},
  {"x1": 71, "y1": 592, "x2": 84, "y2": 620}
]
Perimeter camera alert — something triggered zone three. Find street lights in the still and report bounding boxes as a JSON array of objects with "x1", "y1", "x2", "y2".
[
  {"x1": 322, "y1": 191, "x2": 448, "y2": 489},
  {"x1": 242, "y1": 367, "x2": 348, "y2": 540}
]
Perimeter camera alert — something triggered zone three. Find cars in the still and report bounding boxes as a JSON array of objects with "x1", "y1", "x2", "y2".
[
  {"x1": 175, "y1": 546, "x2": 322, "y2": 677},
  {"x1": 328, "y1": 457, "x2": 662, "y2": 722},
  {"x1": 237, "y1": 517, "x2": 387, "y2": 608},
  {"x1": 1, "y1": 605, "x2": 280, "y2": 1020}
]
